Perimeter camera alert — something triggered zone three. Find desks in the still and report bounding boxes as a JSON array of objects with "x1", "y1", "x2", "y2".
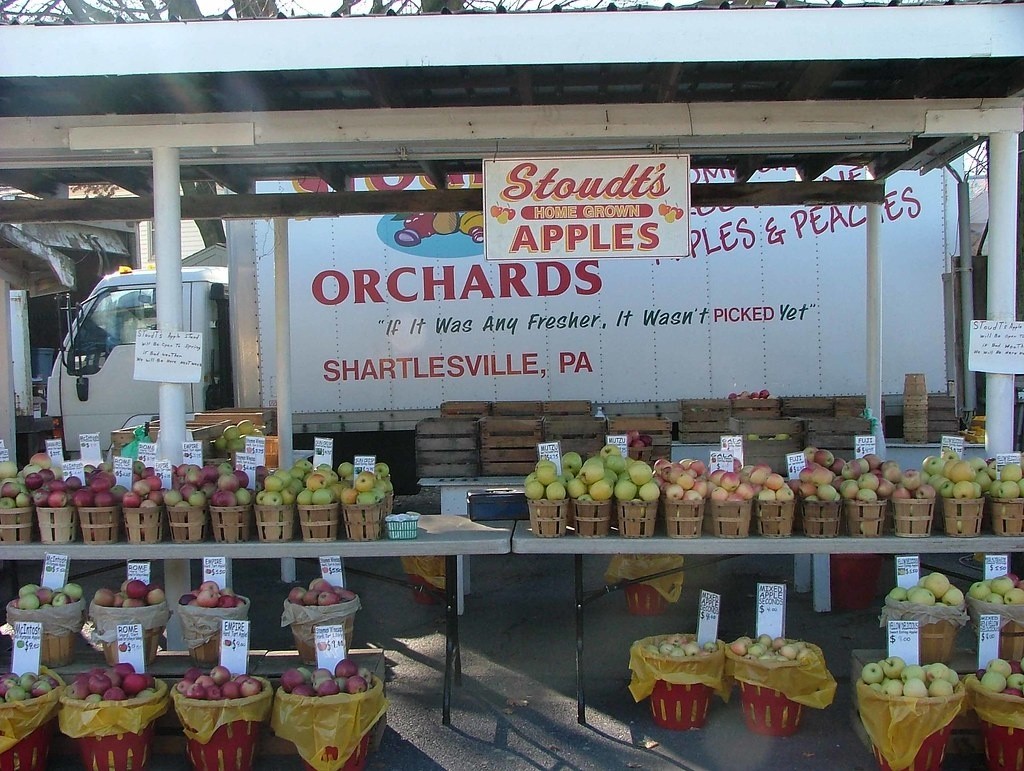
[
  {"x1": 1, "y1": 513, "x2": 517, "y2": 726},
  {"x1": 512, "y1": 519, "x2": 1024, "y2": 724}
]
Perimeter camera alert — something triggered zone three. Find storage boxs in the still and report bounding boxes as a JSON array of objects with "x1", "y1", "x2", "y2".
[
  {"x1": 108, "y1": 406, "x2": 276, "y2": 465},
  {"x1": 414, "y1": 389, "x2": 871, "y2": 477}
]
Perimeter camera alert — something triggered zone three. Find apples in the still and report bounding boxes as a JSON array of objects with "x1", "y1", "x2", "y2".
[
  {"x1": 11, "y1": 577, "x2": 245, "y2": 610},
  {"x1": 288, "y1": 579, "x2": 356, "y2": 606},
  {"x1": 0, "y1": 420, "x2": 392, "y2": 523},
  {"x1": 282, "y1": 659, "x2": 373, "y2": 697},
  {"x1": 644, "y1": 634, "x2": 718, "y2": 657},
  {"x1": 0, "y1": 663, "x2": 159, "y2": 705},
  {"x1": 862, "y1": 655, "x2": 1024, "y2": 699},
  {"x1": 524, "y1": 429, "x2": 1024, "y2": 532},
  {"x1": 730, "y1": 633, "x2": 813, "y2": 662},
  {"x1": 175, "y1": 665, "x2": 262, "y2": 700},
  {"x1": 890, "y1": 571, "x2": 1024, "y2": 608},
  {"x1": 727, "y1": 389, "x2": 776, "y2": 411}
]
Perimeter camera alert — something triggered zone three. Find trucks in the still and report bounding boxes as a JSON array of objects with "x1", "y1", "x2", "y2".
[{"x1": 44, "y1": 159, "x2": 958, "y2": 462}]
